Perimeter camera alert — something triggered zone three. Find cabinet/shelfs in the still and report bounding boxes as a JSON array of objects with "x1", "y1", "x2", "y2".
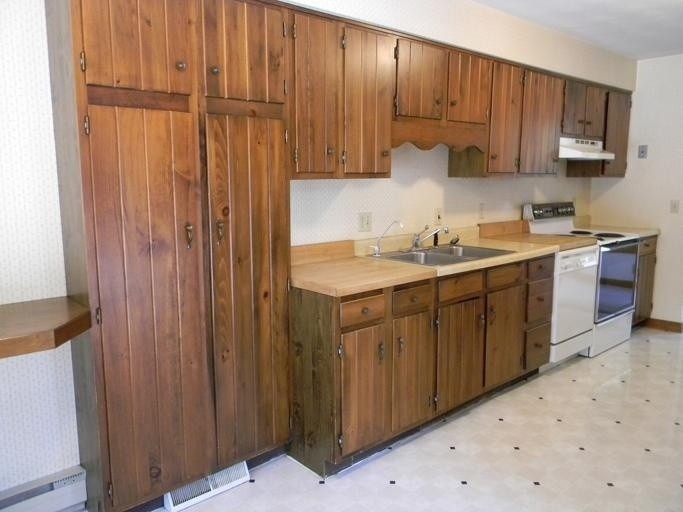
[
  {"x1": 563, "y1": 75, "x2": 607, "y2": 142},
  {"x1": 568, "y1": 87, "x2": 631, "y2": 179},
  {"x1": 43, "y1": 1, "x2": 291, "y2": 105},
  {"x1": 291, "y1": 273, "x2": 437, "y2": 476},
  {"x1": 46, "y1": 105, "x2": 294, "y2": 511},
  {"x1": 437, "y1": 260, "x2": 523, "y2": 417},
  {"x1": 447, "y1": 59, "x2": 563, "y2": 180},
  {"x1": 634, "y1": 235, "x2": 661, "y2": 329},
  {"x1": 392, "y1": 35, "x2": 493, "y2": 154},
  {"x1": 523, "y1": 252, "x2": 555, "y2": 375},
  {"x1": 290, "y1": 2, "x2": 392, "y2": 179}
]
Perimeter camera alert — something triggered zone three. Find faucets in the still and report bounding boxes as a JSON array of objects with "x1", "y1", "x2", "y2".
[
  {"x1": 414, "y1": 224, "x2": 449, "y2": 249},
  {"x1": 368, "y1": 220, "x2": 405, "y2": 257}
]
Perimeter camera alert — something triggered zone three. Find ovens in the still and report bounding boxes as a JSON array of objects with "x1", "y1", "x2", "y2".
[{"x1": 592, "y1": 242, "x2": 639, "y2": 356}]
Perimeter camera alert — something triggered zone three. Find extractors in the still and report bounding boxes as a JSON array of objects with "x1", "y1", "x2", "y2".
[{"x1": 556, "y1": 136, "x2": 615, "y2": 163}]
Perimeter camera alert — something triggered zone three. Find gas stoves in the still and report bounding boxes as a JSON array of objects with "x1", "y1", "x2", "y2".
[{"x1": 552, "y1": 229, "x2": 637, "y2": 244}]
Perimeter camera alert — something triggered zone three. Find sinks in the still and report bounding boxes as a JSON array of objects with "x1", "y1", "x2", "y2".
[
  {"x1": 387, "y1": 252, "x2": 466, "y2": 266},
  {"x1": 430, "y1": 245, "x2": 504, "y2": 259}
]
